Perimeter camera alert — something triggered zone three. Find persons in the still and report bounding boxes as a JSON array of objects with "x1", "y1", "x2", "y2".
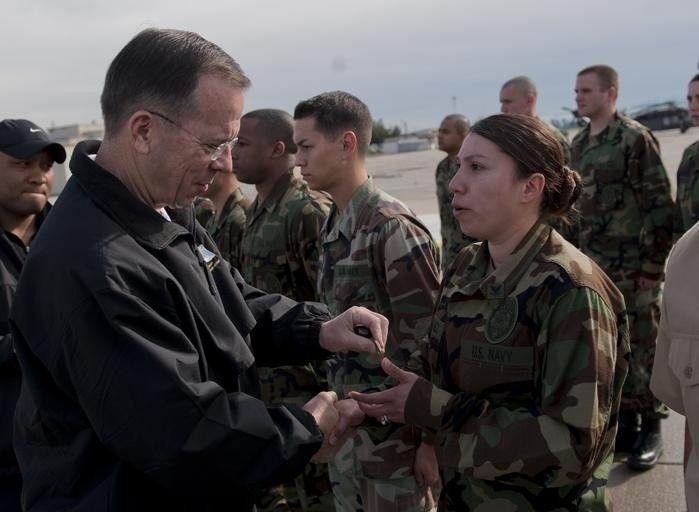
[
  {"x1": 436, "y1": 113, "x2": 477, "y2": 265},
  {"x1": 570, "y1": 65, "x2": 677, "y2": 467},
  {"x1": 293, "y1": 91, "x2": 441, "y2": 512},
  {"x1": 651, "y1": 221, "x2": 699, "y2": 512},
  {"x1": 201, "y1": 108, "x2": 332, "y2": 512},
  {"x1": 500, "y1": 76, "x2": 571, "y2": 166},
  {"x1": 676, "y1": 73, "x2": 699, "y2": 236},
  {"x1": 10, "y1": 28, "x2": 389, "y2": 512},
  {"x1": 350, "y1": 113, "x2": 630, "y2": 512},
  {"x1": 0, "y1": 118, "x2": 67, "y2": 512}
]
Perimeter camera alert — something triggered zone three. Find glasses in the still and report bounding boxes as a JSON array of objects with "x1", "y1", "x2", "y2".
[{"x1": 146, "y1": 108, "x2": 239, "y2": 162}]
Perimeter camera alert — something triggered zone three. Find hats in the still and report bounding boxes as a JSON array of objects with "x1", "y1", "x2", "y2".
[{"x1": 1, "y1": 118, "x2": 66, "y2": 164}]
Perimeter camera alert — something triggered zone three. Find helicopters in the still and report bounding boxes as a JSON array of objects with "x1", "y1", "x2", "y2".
[{"x1": 563, "y1": 98, "x2": 696, "y2": 137}]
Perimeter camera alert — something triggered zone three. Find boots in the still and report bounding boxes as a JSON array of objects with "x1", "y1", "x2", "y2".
[{"x1": 626, "y1": 430, "x2": 664, "y2": 470}]
